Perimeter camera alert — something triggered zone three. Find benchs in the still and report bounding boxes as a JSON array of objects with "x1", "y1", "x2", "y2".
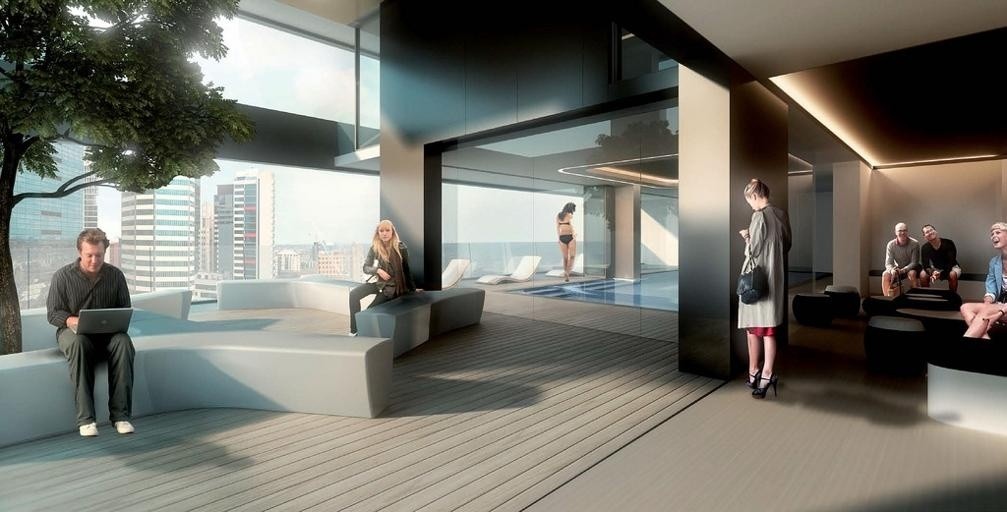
[
  {"x1": 19, "y1": 286, "x2": 194, "y2": 352},
  {"x1": 902, "y1": 286, "x2": 963, "y2": 311},
  {"x1": 216, "y1": 275, "x2": 433, "y2": 360},
  {"x1": 297, "y1": 273, "x2": 486, "y2": 339},
  {"x1": 894, "y1": 305, "x2": 1007, "y2": 378},
  {"x1": 869, "y1": 269, "x2": 988, "y2": 284},
  {"x1": 0, "y1": 290, "x2": 394, "y2": 447}
]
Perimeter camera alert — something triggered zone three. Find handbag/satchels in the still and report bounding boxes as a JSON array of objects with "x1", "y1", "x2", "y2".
[
  {"x1": 736, "y1": 265, "x2": 769, "y2": 304},
  {"x1": 887, "y1": 270, "x2": 904, "y2": 297}
]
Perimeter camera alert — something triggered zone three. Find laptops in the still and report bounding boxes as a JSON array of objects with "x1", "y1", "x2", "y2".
[{"x1": 69, "y1": 308, "x2": 133, "y2": 335}]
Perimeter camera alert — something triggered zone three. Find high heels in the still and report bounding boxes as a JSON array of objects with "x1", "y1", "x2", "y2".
[
  {"x1": 752, "y1": 373, "x2": 778, "y2": 398},
  {"x1": 745, "y1": 369, "x2": 762, "y2": 389}
]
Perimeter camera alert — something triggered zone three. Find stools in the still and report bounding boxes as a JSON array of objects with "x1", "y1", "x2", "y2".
[
  {"x1": 823, "y1": 285, "x2": 862, "y2": 319},
  {"x1": 863, "y1": 315, "x2": 927, "y2": 375},
  {"x1": 862, "y1": 295, "x2": 897, "y2": 317},
  {"x1": 792, "y1": 293, "x2": 832, "y2": 326}
]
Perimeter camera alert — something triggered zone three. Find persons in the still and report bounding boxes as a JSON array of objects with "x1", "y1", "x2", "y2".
[
  {"x1": 921, "y1": 225, "x2": 963, "y2": 291},
  {"x1": 882, "y1": 223, "x2": 920, "y2": 296},
  {"x1": 349, "y1": 221, "x2": 424, "y2": 336},
  {"x1": 736, "y1": 179, "x2": 793, "y2": 398},
  {"x1": 556, "y1": 202, "x2": 577, "y2": 280},
  {"x1": 44, "y1": 227, "x2": 136, "y2": 436},
  {"x1": 959, "y1": 221, "x2": 1007, "y2": 339}
]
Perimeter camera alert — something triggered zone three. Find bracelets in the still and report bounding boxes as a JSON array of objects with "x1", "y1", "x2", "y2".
[
  {"x1": 999, "y1": 309, "x2": 1005, "y2": 316},
  {"x1": 743, "y1": 233, "x2": 749, "y2": 240}
]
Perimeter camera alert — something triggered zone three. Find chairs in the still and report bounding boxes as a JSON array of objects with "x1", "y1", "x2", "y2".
[
  {"x1": 547, "y1": 252, "x2": 586, "y2": 278},
  {"x1": 442, "y1": 258, "x2": 471, "y2": 288},
  {"x1": 477, "y1": 254, "x2": 542, "y2": 286}
]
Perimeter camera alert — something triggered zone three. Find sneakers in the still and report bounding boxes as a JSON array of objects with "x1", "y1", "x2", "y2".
[
  {"x1": 115, "y1": 421, "x2": 135, "y2": 434},
  {"x1": 79, "y1": 422, "x2": 99, "y2": 436}
]
